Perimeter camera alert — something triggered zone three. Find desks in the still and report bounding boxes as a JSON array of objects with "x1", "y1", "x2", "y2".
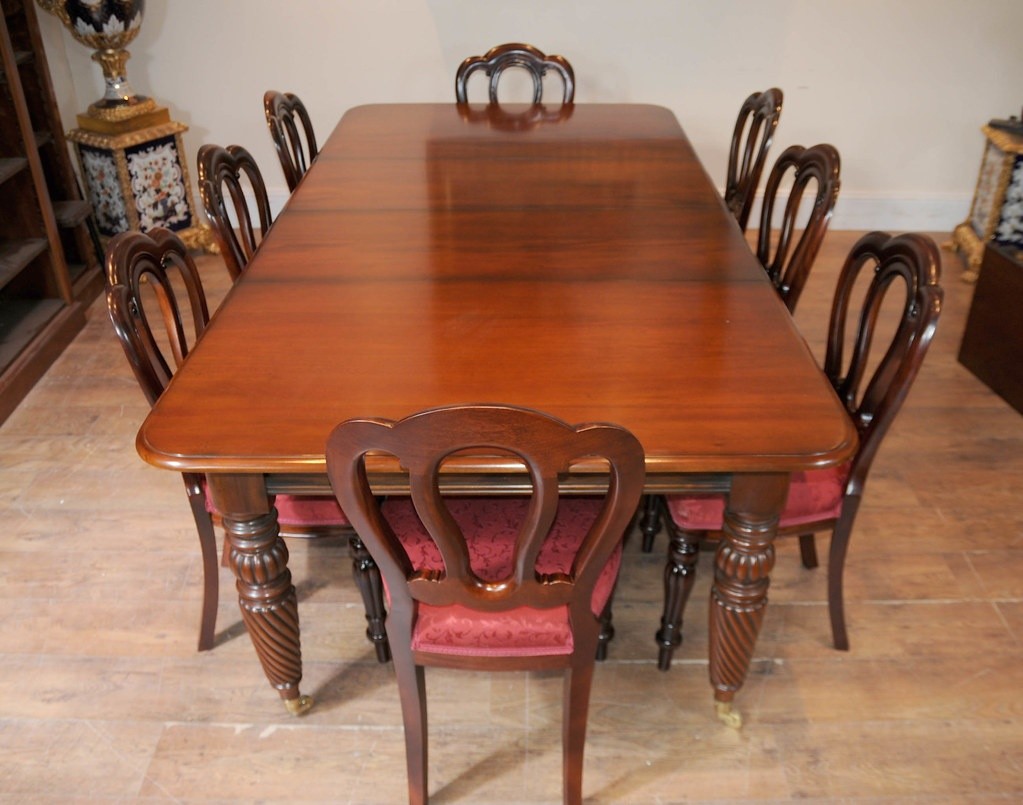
[{"x1": 135, "y1": 102, "x2": 858, "y2": 732}]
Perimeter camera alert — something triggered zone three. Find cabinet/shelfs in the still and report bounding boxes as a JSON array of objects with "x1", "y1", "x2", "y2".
[{"x1": 0, "y1": 0, "x2": 111, "y2": 429}]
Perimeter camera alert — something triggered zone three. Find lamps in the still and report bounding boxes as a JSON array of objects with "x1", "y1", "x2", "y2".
[
  {"x1": 943, "y1": 106, "x2": 1023, "y2": 283},
  {"x1": 36, "y1": 0, "x2": 225, "y2": 283}
]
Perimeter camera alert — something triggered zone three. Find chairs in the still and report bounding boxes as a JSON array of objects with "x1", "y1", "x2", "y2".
[
  {"x1": 263, "y1": 90, "x2": 318, "y2": 194},
  {"x1": 455, "y1": 42, "x2": 576, "y2": 104},
  {"x1": 325, "y1": 401, "x2": 646, "y2": 805},
  {"x1": 641, "y1": 230, "x2": 943, "y2": 671},
  {"x1": 754, "y1": 143, "x2": 841, "y2": 315},
  {"x1": 724, "y1": 87, "x2": 784, "y2": 235},
  {"x1": 195, "y1": 142, "x2": 270, "y2": 283},
  {"x1": 105, "y1": 226, "x2": 394, "y2": 664}
]
